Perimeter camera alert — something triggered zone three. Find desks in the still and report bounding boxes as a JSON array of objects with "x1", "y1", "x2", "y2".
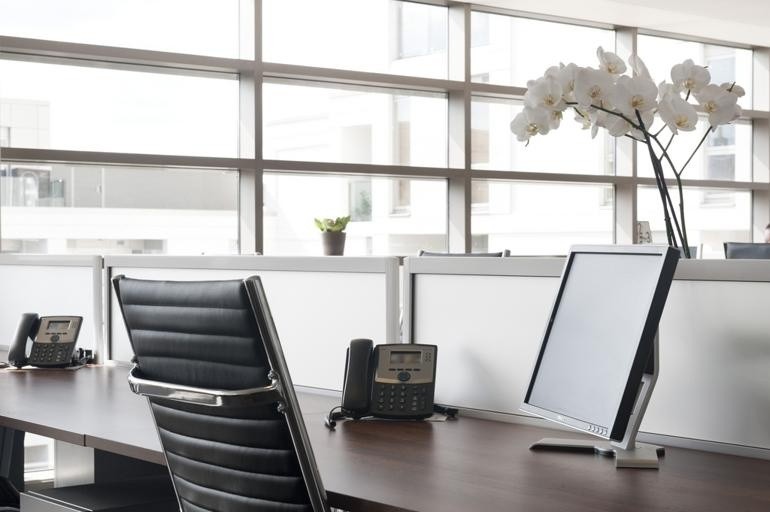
[{"x1": 2, "y1": 358, "x2": 768, "y2": 512}]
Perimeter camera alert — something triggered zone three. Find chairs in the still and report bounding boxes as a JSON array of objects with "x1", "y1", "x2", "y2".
[{"x1": 111, "y1": 275, "x2": 332, "y2": 512}]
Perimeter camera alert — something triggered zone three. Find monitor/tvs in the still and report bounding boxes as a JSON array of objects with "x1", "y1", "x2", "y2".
[{"x1": 518, "y1": 242, "x2": 681, "y2": 469}]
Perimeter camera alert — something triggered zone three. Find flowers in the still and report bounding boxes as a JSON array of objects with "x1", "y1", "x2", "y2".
[{"x1": 511, "y1": 46, "x2": 746, "y2": 260}]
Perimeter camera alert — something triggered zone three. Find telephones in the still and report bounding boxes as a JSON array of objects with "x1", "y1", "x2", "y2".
[
  {"x1": 7, "y1": 313, "x2": 83, "y2": 368},
  {"x1": 341, "y1": 339, "x2": 438, "y2": 421}
]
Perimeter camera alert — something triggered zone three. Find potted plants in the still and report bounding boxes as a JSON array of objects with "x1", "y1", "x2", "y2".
[{"x1": 314, "y1": 216, "x2": 351, "y2": 255}]
